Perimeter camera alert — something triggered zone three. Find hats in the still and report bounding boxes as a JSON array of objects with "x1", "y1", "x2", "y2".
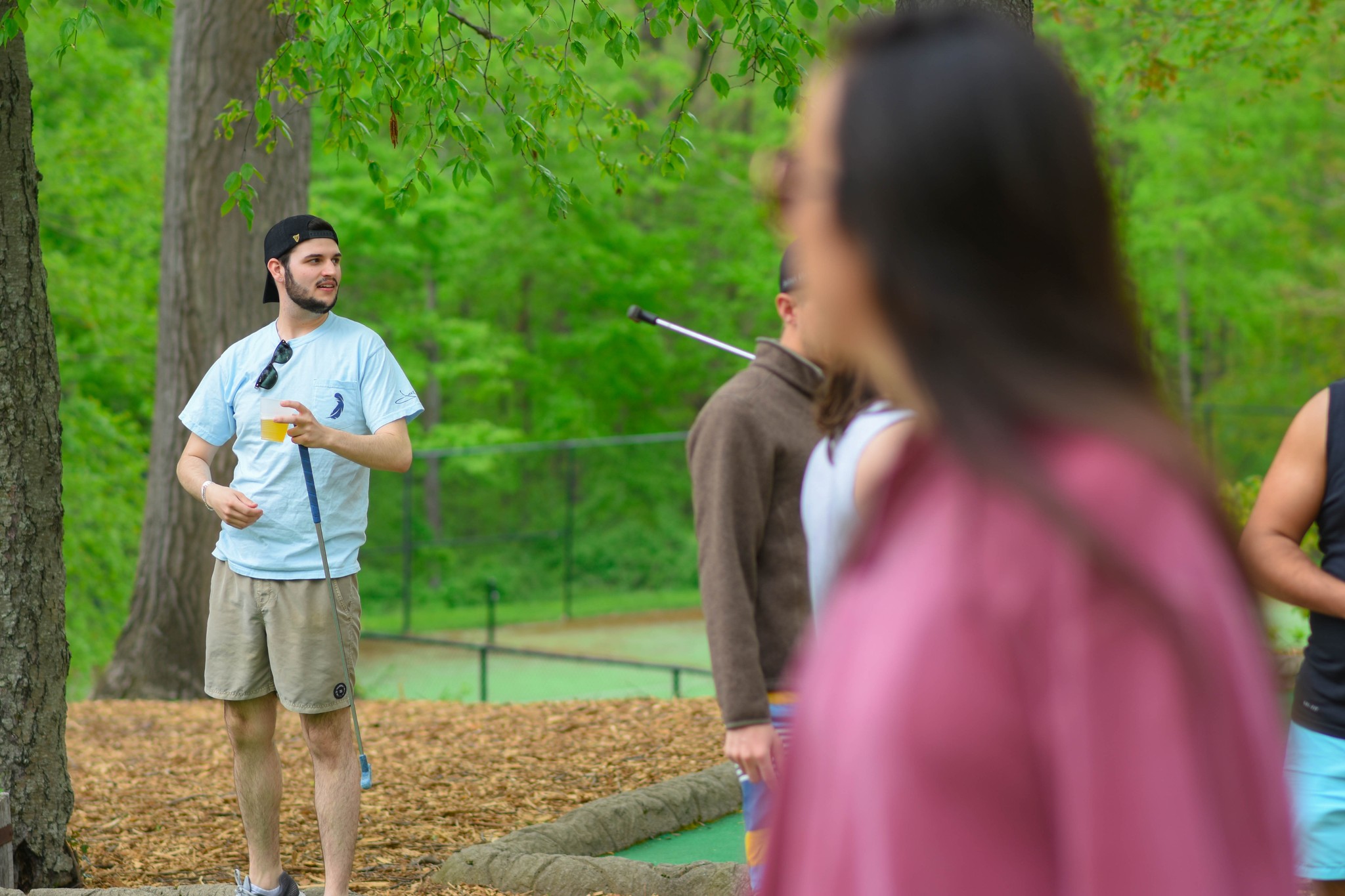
[{"x1": 263, "y1": 215, "x2": 338, "y2": 303}]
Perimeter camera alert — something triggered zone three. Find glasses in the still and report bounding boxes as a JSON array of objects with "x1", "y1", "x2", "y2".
[{"x1": 255, "y1": 339, "x2": 293, "y2": 390}]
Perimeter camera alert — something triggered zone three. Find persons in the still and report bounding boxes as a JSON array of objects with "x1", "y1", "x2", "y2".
[
  {"x1": 175, "y1": 211, "x2": 426, "y2": 896},
  {"x1": 683, "y1": 12, "x2": 1298, "y2": 895},
  {"x1": 1239, "y1": 376, "x2": 1345, "y2": 895}
]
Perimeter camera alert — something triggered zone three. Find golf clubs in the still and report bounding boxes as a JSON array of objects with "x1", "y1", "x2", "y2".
[
  {"x1": 294, "y1": 423, "x2": 373, "y2": 789},
  {"x1": 627, "y1": 304, "x2": 757, "y2": 361}
]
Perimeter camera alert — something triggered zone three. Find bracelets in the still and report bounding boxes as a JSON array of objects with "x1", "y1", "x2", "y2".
[{"x1": 201, "y1": 480, "x2": 214, "y2": 511}]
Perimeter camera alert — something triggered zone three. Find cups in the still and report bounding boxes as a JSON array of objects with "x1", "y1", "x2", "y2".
[{"x1": 260, "y1": 398, "x2": 294, "y2": 442}]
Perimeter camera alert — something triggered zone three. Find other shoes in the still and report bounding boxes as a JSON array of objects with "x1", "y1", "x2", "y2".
[{"x1": 234, "y1": 868, "x2": 306, "y2": 896}]
